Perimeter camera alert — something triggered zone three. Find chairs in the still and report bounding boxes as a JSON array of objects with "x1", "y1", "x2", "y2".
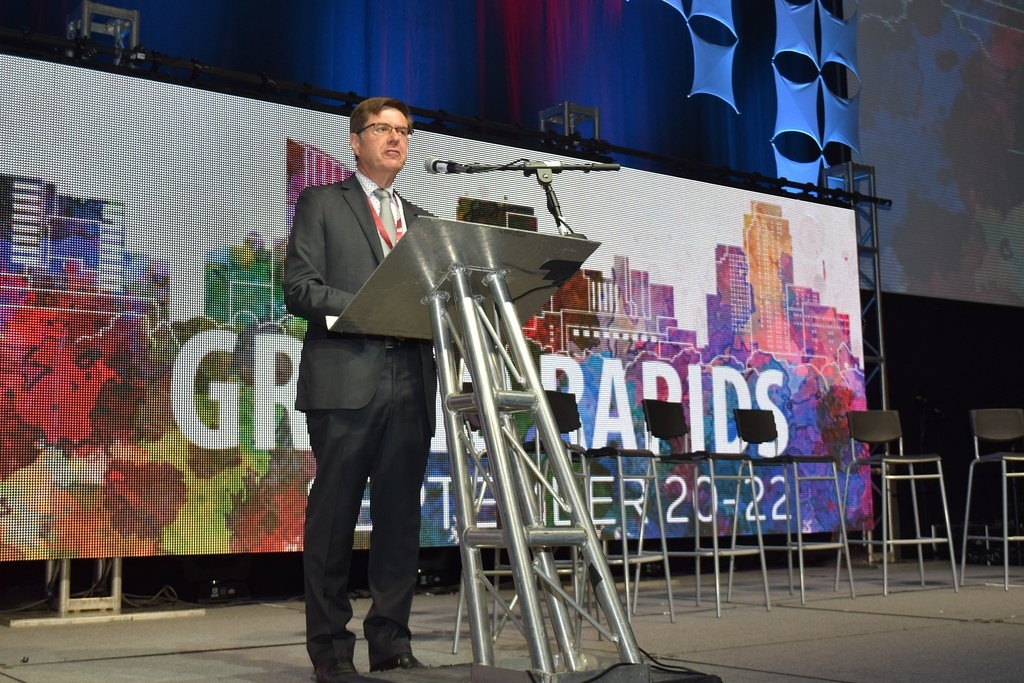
[
  {"x1": 633, "y1": 398, "x2": 771, "y2": 618},
  {"x1": 451, "y1": 382, "x2": 584, "y2": 659},
  {"x1": 834, "y1": 410, "x2": 959, "y2": 601},
  {"x1": 725, "y1": 408, "x2": 858, "y2": 607},
  {"x1": 960, "y1": 407, "x2": 1024, "y2": 590},
  {"x1": 534, "y1": 390, "x2": 678, "y2": 627}
]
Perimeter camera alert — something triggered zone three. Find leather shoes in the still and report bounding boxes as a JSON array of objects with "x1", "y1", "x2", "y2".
[
  {"x1": 369, "y1": 653, "x2": 427, "y2": 672},
  {"x1": 315, "y1": 657, "x2": 361, "y2": 683}
]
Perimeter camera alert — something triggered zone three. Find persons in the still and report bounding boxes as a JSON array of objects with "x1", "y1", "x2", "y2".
[{"x1": 283, "y1": 98, "x2": 437, "y2": 683}]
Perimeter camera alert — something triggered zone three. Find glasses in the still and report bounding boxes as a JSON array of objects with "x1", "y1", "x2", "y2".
[{"x1": 356, "y1": 122, "x2": 414, "y2": 139}]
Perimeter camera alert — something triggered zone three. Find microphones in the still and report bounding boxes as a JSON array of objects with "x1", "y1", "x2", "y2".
[{"x1": 425, "y1": 157, "x2": 471, "y2": 174}]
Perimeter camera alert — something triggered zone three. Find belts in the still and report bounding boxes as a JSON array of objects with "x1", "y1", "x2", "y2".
[{"x1": 385, "y1": 336, "x2": 416, "y2": 351}]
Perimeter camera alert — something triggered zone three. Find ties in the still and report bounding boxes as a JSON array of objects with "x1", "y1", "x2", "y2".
[{"x1": 373, "y1": 189, "x2": 396, "y2": 258}]
